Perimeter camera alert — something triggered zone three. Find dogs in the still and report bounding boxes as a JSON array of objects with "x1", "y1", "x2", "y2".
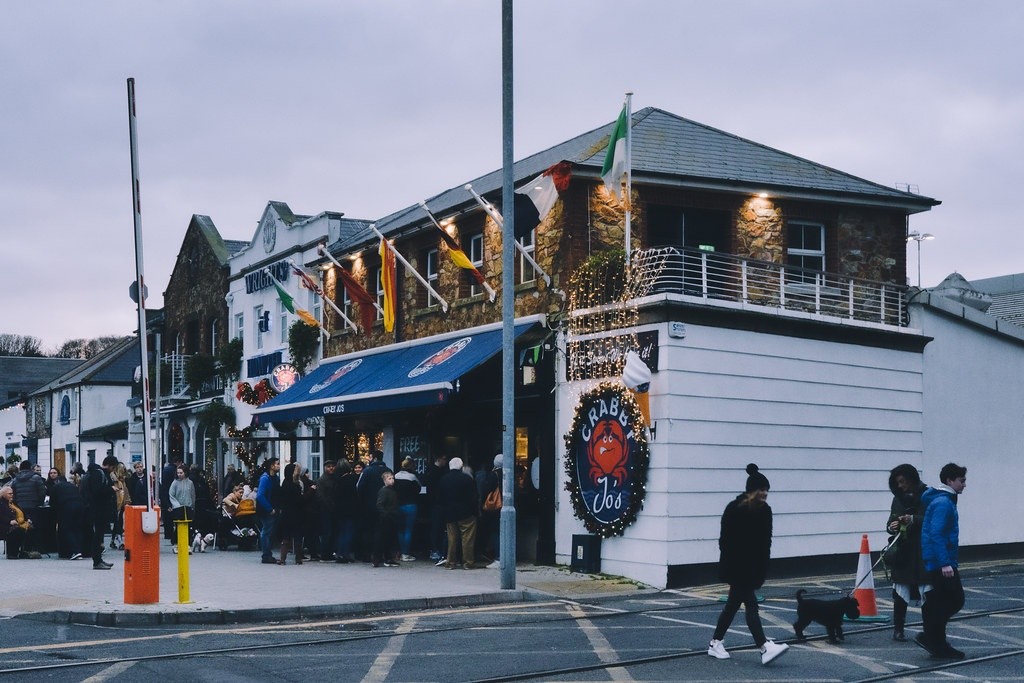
[
  {"x1": 794, "y1": 587, "x2": 860, "y2": 645},
  {"x1": 191, "y1": 532, "x2": 215, "y2": 554}
]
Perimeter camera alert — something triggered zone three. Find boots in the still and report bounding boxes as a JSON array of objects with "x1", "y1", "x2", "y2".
[{"x1": 892, "y1": 588, "x2": 910, "y2": 641}]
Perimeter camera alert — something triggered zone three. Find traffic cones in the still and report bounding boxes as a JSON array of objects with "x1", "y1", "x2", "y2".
[{"x1": 841, "y1": 535, "x2": 892, "y2": 624}]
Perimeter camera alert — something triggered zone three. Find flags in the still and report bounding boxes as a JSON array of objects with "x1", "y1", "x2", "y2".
[
  {"x1": 290, "y1": 263, "x2": 325, "y2": 300},
  {"x1": 426, "y1": 209, "x2": 486, "y2": 286},
  {"x1": 318, "y1": 244, "x2": 375, "y2": 335},
  {"x1": 599, "y1": 98, "x2": 628, "y2": 206},
  {"x1": 267, "y1": 272, "x2": 322, "y2": 327},
  {"x1": 377, "y1": 236, "x2": 400, "y2": 332},
  {"x1": 471, "y1": 163, "x2": 571, "y2": 237}
]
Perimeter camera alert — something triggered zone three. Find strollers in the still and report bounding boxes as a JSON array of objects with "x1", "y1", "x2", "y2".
[{"x1": 217, "y1": 498, "x2": 263, "y2": 552}]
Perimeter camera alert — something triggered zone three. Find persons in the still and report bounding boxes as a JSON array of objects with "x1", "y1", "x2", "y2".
[
  {"x1": 161, "y1": 457, "x2": 186, "y2": 539},
  {"x1": 920, "y1": 460, "x2": 968, "y2": 658},
  {"x1": 887, "y1": 462, "x2": 933, "y2": 641},
  {"x1": 253, "y1": 449, "x2": 533, "y2": 569},
  {"x1": 169, "y1": 465, "x2": 197, "y2": 555},
  {"x1": 0, "y1": 456, "x2": 164, "y2": 570},
  {"x1": 187, "y1": 463, "x2": 210, "y2": 499},
  {"x1": 222, "y1": 465, "x2": 244, "y2": 498},
  {"x1": 707, "y1": 463, "x2": 789, "y2": 665},
  {"x1": 222, "y1": 482, "x2": 244, "y2": 518}
]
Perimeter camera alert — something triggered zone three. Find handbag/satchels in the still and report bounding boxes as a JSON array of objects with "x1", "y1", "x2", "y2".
[
  {"x1": 883, "y1": 535, "x2": 910, "y2": 568},
  {"x1": 483, "y1": 471, "x2": 504, "y2": 511}
]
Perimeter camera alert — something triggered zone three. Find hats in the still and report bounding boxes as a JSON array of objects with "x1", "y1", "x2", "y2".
[
  {"x1": 744, "y1": 464, "x2": 771, "y2": 492},
  {"x1": 494, "y1": 454, "x2": 504, "y2": 469},
  {"x1": 449, "y1": 457, "x2": 465, "y2": 470},
  {"x1": 324, "y1": 459, "x2": 336, "y2": 465}
]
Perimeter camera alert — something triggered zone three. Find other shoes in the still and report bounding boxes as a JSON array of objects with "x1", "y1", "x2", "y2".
[
  {"x1": 445, "y1": 561, "x2": 459, "y2": 569},
  {"x1": 261, "y1": 550, "x2": 371, "y2": 566},
  {"x1": 913, "y1": 632, "x2": 948, "y2": 656},
  {"x1": 486, "y1": 559, "x2": 500, "y2": 568},
  {"x1": 400, "y1": 553, "x2": 417, "y2": 561},
  {"x1": 172, "y1": 545, "x2": 179, "y2": 554},
  {"x1": 372, "y1": 559, "x2": 384, "y2": 567},
  {"x1": 384, "y1": 559, "x2": 400, "y2": 567},
  {"x1": 429, "y1": 550, "x2": 448, "y2": 560},
  {"x1": 930, "y1": 643, "x2": 965, "y2": 660},
  {"x1": 69, "y1": 542, "x2": 124, "y2": 569},
  {"x1": 188, "y1": 545, "x2": 191, "y2": 554},
  {"x1": 464, "y1": 562, "x2": 476, "y2": 569},
  {"x1": 436, "y1": 556, "x2": 448, "y2": 566}
]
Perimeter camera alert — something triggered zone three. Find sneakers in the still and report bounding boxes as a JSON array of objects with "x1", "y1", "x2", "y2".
[
  {"x1": 708, "y1": 639, "x2": 731, "y2": 659},
  {"x1": 761, "y1": 639, "x2": 789, "y2": 665}
]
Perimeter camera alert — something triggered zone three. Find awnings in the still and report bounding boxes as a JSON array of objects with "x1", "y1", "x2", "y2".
[{"x1": 249, "y1": 311, "x2": 547, "y2": 427}]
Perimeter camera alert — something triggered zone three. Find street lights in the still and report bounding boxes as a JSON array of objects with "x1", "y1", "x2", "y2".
[{"x1": 907, "y1": 232, "x2": 935, "y2": 287}]
